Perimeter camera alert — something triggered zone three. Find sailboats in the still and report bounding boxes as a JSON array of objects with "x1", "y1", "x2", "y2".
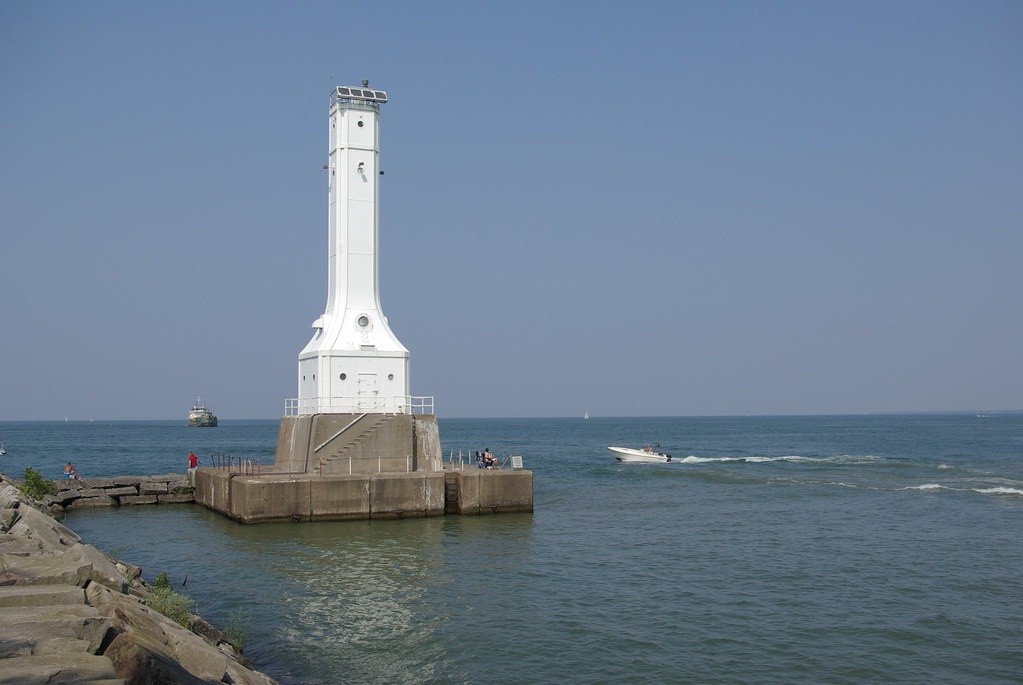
[{"x1": 583, "y1": 411, "x2": 590, "y2": 419}]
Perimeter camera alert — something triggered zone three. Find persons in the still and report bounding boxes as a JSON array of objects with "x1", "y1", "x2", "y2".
[
  {"x1": 64, "y1": 462, "x2": 75, "y2": 472},
  {"x1": 645, "y1": 443, "x2": 653, "y2": 452},
  {"x1": 484, "y1": 448, "x2": 499, "y2": 467},
  {"x1": 187, "y1": 451, "x2": 199, "y2": 476}
]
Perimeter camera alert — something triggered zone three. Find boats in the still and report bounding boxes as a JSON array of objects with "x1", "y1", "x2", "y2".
[
  {"x1": 608, "y1": 447, "x2": 668, "y2": 463},
  {"x1": 188, "y1": 396, "x2": 217, "y2": 428}
]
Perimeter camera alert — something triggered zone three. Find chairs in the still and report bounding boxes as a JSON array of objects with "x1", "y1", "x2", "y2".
[{"x1": 475, "y1": 451, "x2": 498, "y2": 471}]
